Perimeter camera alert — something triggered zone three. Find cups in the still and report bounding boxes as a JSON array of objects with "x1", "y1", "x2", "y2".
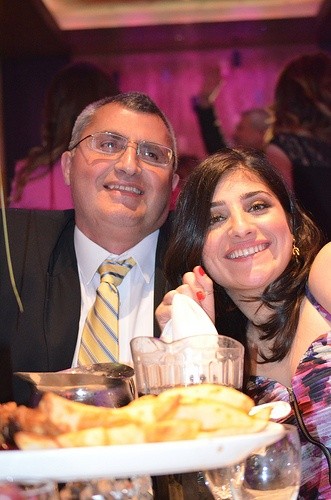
[
  {"x1": 229, "y1": 424, "x2": 302, "y2": 500},
  {"x1": 205, "y1": 463, "x2": 243, "y2": 500}
]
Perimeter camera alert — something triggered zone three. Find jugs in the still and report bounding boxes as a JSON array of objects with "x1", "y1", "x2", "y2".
[
  {"x1": 130, "y1": 334, "x2": 243, "y2": 500},
  {"x1": 15, "y1": 363, "x2": 153, "y2": 500}
]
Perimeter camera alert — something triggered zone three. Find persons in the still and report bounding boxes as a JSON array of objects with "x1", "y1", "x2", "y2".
[{"x1": 0, "y1": 52, "x2": 331, "y2": 500}]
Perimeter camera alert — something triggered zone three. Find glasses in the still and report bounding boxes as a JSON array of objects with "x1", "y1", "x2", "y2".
[{"x1": 70, "y1": 132, "x2": 177, "y2": 168}]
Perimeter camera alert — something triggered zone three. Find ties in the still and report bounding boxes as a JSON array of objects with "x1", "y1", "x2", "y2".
[{"x1": 77, "y1": 256, "x2": 135, "y2": 368}]
